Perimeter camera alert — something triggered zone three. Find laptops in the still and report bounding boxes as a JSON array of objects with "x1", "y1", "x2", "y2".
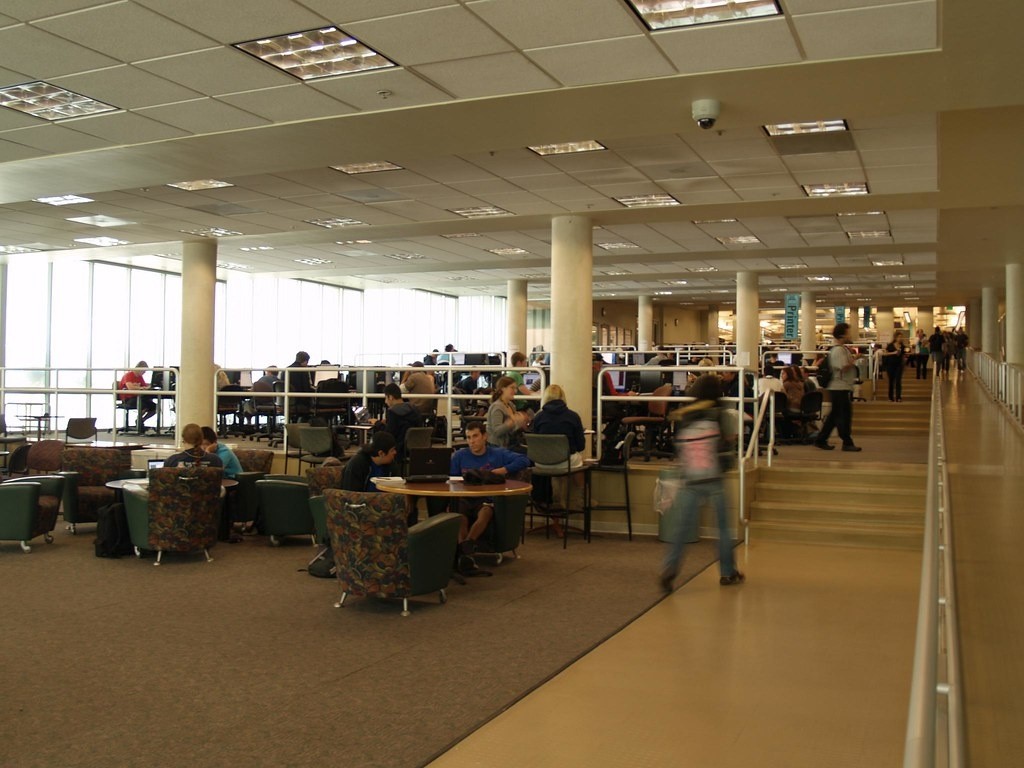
[
  {"x1": 147, "y1": 459, "x2": 166, "y2": 469},
  {"x1": 407, "y1": 448, "x2": 453, "y2": 478},
  {"x1": 352, "y1": 405, "x2": 375, "y2": 425}
]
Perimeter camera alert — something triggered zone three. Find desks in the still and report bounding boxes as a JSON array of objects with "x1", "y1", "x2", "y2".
[
  {"x1": 17, "y1": 414, "x2": 67, "y2": 442},
  {"x1": 376, "y1": 476, "x2": 534, "y2": 585},
  {"x1": 104, "y1": 479, "x2": 240, "y2": 504}
]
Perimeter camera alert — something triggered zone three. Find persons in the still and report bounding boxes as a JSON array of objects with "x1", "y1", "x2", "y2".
[
  {"x1": 505, "y1": 352, "x2": 541, "y2": 414},
  {"x1": 454, "y1": 370, "x2": 600, "y2": 512},
  {"x1": 811, "y1": 322, "x2": 864, "y2": 452},
  {"x1": 164, "y1": 424, "x2": 221, "y2": 470},
  {"x1": 655, "y1": 375, "x2": 746, "y2": 592},
  {"x1": 120, "y1": 360, "x2": 156, "y2": 434},
  {"x1": 779, "y1": 363, "x2": 818, "y2": 438},
  {"x1": 521, "y1": 339, "x2": 819, "y2": 398},
  {"x1": 339, "y1": 430, "x2": 398, "y2": 492},
  {"x1": 201, "y1": 426, "x2": 244, "y2": 480},
  {"x1": 243, "y1": 352, "x2": 359, "y2": 452},
  {"x1": 368, "y1": 383, "x2": 423, "y2": 446},
  {"x1": 591, "y1": 347, "x2": 640, "y2": 465},
  {"x1": 399, "y1": 344, "x2": 464, "y2": 407},
  {"x1": 213, "y1": 363, "x2": 230, "y2": 390},
  {"x1": 446, "y1": 421, "x2": 530, "y2": 571},
  {"x1": 752, "y1": 366, "x2": 788, "y2": 437},
  {"x1": 872, "y1": 324, "x2": 971, "y2": 404}
]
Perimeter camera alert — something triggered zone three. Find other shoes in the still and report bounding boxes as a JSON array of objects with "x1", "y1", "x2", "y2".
[
  {"x1": 454, "y1": 547, "x2": 477, "y2": 572},
  {"x1": 663, "y1": 581, "x2": 672, "y2": 591},
  {"x1": 719, "y1": 573, "x2": 745, "y2": 586},
  {"x1": 889, "y1": 399, "x2": 894, "y2": 401},
  {"x1": 896, "y1": 398, "x2": 901, "y2": 401}
]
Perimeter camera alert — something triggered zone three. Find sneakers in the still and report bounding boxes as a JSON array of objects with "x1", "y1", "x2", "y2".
[
  {"x1": 815, "y1": 439, "x2": 834, "y2": 450},
  {"x1": 842, "y1": 442, "x2": 862, "y2": 451}
]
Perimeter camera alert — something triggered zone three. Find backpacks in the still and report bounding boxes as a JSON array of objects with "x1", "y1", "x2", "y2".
[
  {"x1": 427, "y1": 415, "x2": 447, "y2": 438},
  {"x1": 310, "y1": 417, "x2": 343, "y2": 456},
  {"x1": 817, "y1": 345, "x2": 840, "y2": 388},
  {"x1": 308, "y1": 546, "x2": 337, "y2": 578},
  {"x1": 93, "y1": 489, "x2": 132, "y2": 558}
]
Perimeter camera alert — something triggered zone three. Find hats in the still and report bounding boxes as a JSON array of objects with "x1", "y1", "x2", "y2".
[
  {"x1": 593, "y1": 353, "x2": 608, "y2": 363},
  {"x1": 408, "y1": 362, "x2": 423, "y2": 367}
]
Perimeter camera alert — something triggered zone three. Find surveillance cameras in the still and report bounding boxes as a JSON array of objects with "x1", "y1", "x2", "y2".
[{"x1": 691, "y1": 99, "x2": 720, "y2": 130}]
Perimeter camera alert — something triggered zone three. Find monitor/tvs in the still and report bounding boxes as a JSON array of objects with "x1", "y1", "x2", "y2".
[
  {"x1": 314, "y1": 366, "x2": 339, "y2": 387},
  {"x1": 778, "y1": 353, "x2": 791, "y2": 366},
  {"x1": 522, "y1": 373, "x2": 540, "y2": 389},
  {"x1": 602, "y1": 353, "x2": 630, "y2": 392},
  {"x1": 151, "y1": 366, "x2": 179, "y2": 390}
]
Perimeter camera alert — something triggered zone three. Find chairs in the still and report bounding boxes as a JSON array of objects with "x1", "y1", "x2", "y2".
[{"x1": 0, "y1": 380, "x2": 867, "y2": 619}]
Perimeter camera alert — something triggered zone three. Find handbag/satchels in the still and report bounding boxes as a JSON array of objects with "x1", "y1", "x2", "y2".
[
  {"x1": 317, "y1": 378, "x2": 354, "y2": 405},
  {"x1": 601, "y1": 431, "x2": 626, "y2": 463},
  {"x1": 233, "y1": 521, "x2": 258, "y2": 536},
  {"x1": 674, "y1": 418, "x2": 722, "y2": 485}
]
